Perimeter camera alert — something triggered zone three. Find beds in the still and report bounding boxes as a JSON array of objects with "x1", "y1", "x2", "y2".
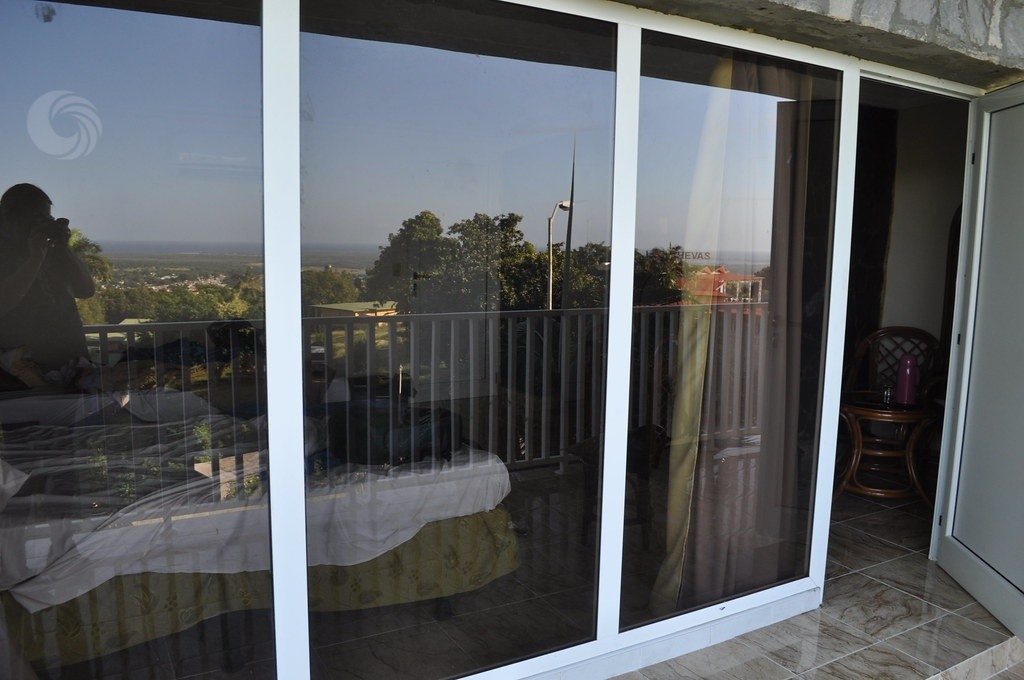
[{"x1": 0, "y1": 413, "x2": 522, "y2": 669}]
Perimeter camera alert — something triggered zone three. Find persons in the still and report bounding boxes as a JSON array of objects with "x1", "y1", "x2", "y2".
[{"x1": 1, "y1": 184, "x2": 95, "y2": 585}]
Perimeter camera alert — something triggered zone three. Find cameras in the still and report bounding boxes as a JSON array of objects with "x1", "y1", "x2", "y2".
[{"x1": 40, "y1": 225, "x2": 62, "y2": 247}]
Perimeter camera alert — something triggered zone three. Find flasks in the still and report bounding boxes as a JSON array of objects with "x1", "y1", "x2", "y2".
[{"x1": 896, "y1": 353, "x2": 919, "y2": 405}]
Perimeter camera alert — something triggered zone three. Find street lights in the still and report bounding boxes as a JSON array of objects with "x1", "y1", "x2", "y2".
[{"x1": 545, "y1": 200, "x2": 574, "y2": 310}]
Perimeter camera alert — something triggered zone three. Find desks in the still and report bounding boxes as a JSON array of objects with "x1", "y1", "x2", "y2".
[{"x1": 833, "y1": 389, "x2": 944, "y2": 508}]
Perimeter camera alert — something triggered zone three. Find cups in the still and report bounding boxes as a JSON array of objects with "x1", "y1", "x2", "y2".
[{"x1": 879, "y1": 384, "x2": 896, "y2": 403}]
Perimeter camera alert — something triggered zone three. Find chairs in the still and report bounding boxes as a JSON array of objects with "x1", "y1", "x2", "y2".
[
  {"x1": 566, "y1": 422, "x2": 670, "y2": 553},
  {"x1": 843, "y1": 325, "x2": 949, "y2": 445}
]
